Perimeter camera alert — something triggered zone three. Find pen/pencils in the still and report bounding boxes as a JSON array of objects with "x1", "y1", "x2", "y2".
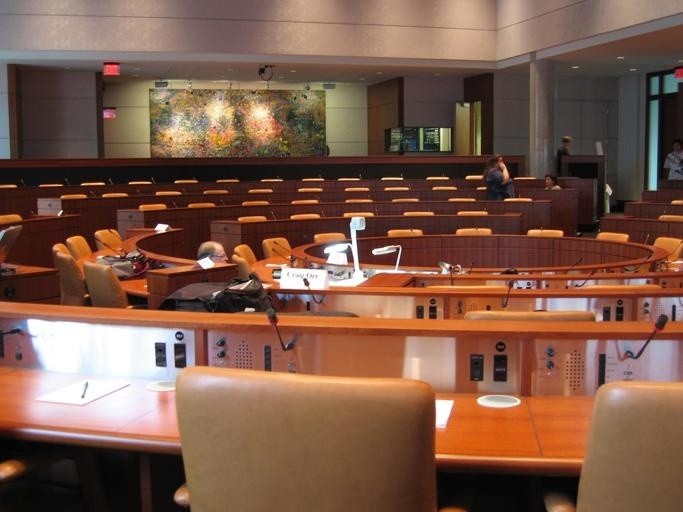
[{"x1": 82, "y1": 381, "x2": 88, "y2": 399}]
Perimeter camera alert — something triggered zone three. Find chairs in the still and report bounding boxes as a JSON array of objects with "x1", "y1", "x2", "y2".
[
  {"x1": 0, "y1": 175, "x2": 683, "y2": 321},
  {"x1": 544, "y1": 380, "x2": 683, "y2": 512},
  {"x1": 174, "y1": 366, "x2": 466, "y2": 511}
]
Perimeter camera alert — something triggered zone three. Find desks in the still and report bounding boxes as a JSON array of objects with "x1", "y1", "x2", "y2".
[{"x1": 2, "y1": 365, "x2": 594, "y2": 474}]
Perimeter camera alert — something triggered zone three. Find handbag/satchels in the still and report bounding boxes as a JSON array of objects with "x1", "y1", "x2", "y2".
[
  {"x1": 207, "y1": 272, "x2": 272, "y2": 312},
  {"x1": 158, "y1": 282, "x2": 229, "y2": 312}
]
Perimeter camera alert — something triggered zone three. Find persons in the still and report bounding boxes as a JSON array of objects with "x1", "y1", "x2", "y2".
[
  {"x1": 543, "y1": 174, "x2": 561, "y2": 190},
  {"x1": 663, "y1": 140, "x2": 683, "y2": 181},
  {"x1": 556, "y1": 136, "x2": 572, "y2": 176},
  {"x1": 197, "y1": 241, "x2": 228, "y2": 264},
  {"x1": 484, "y1": 153, "x2": 515, "y2": 199}
]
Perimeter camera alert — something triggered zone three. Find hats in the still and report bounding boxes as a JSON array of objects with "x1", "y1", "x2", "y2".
[{"x1": 561, "y1": 135, "x2": 571, "y2": 141}]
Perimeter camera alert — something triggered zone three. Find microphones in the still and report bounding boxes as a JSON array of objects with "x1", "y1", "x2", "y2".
[
  {"x1": 272, "y1": 240, "x2": 309, "y2": 269},
  {"x1": 400, "y1": 173, "x2": 413, "y2": 192},
  {"x1": 624, "y1": 254, "x2": 652, "y2": 271},
  {"x1": 359, "y1": 173, "x2": 379, "y2": 216},
  {"x1": 21, "y1": 179, "x2": 26, "y2": 187},
  {"x1": 624, "y1": 314, "x2": 668, "y2": 359},
  {"x1": 575, "y1": 270, "x2": 594, "y2": 287},
  {"x1": 501, "y1": 280, "x2": 514, "y2": 307},
  {"x1": 303, "y1": 278, "x2": 321, "y2": 304},
  {"x1": 469, "y1": 262, "x2": 473, "y2": 274},
  {"x1": 90, "y1": 235, "x2": 126, "y2": 262},
  {"x1": 106, "y1": 226, "x2": 165, "y2": 268},
  {"x1": 90, "y1": 191, "x2": 97, "y2": 199},
  {"x1": 565, "y1": 256, "x2": 583, "y2": 273},
  {"x1": 151, "y1": 177, "x2": 157, "y2": 185},
  {"x1": 172, "y1": 176, "x2": 227, "y2": 208},
  {"x1": 413, "y1": 170, "x2": 545, "y2": 235},
  {"x1": 136, "y1": 188, "x2": 143, "y2": 196},
  {"x1": 644, "y1": 234, "x2": 650, "y2": 246},
  {"x1": 269, "y1": 175, "x2": 280, "y2": 220},
  {"x1": 65, "y1": 178, "x2": 70, "y2": 186},
  {"x1": 108, "y1": 177, "x2": 114, "y2": 185},
  {"x1": 449, "y1": 266, "x2": 453, "y2": 285},
  {"x1": 266, "y1": 307, "x2": 294, "y2": 351},
  {"x1": 315, "y1": 173, "x2": 325, "y2": 217}
]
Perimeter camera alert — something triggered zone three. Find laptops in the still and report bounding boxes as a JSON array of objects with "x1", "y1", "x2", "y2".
[{"x1": 0, "y1": 225, "x2": 23, "y2": 269}]
